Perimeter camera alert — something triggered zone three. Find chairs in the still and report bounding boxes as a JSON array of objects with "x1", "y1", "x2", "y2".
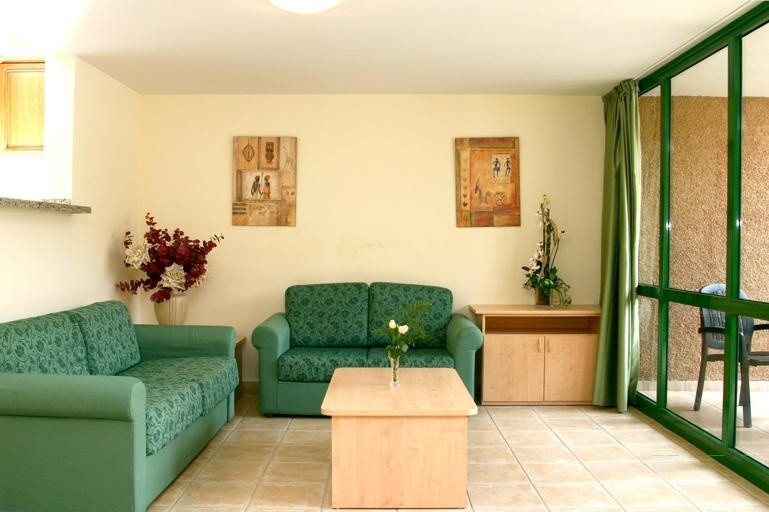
[{"x1": 694, "y1": 283, "x2": 769, "y2": 428}]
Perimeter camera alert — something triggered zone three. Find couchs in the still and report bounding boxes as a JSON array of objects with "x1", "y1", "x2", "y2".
[
  {"x1": 251, "y1": 282, "x2": 484, "y2": 417},
  {"x1": 0, "y1": 300, "x2": 239, "y2": 512}
]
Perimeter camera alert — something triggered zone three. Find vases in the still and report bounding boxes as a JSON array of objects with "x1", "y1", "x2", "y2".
[
  {"x1": 534, "y1": 285, "x2": 550, "y2": 305},
  {"x1": 155, "y1": 294, "x2": 189, "y2": 325},
  {"x1": 388, "y1": 353, "x2": 401, "y2": 387}
]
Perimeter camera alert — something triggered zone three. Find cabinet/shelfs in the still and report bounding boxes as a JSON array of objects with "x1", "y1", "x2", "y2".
[{"x1": 469, "y1": 303, "x2": 602, "y2": 406}]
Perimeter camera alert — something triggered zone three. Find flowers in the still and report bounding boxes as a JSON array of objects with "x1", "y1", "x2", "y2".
[
  {"x1": 116, "y1": 211, "x2": 224, "y2": 303},
  {"x1": 519, "y1": 192, "x2": 571, "y2": 309},
  {"x1": 375, "y1": 301, "x2": 431, "y2": 381}
]
